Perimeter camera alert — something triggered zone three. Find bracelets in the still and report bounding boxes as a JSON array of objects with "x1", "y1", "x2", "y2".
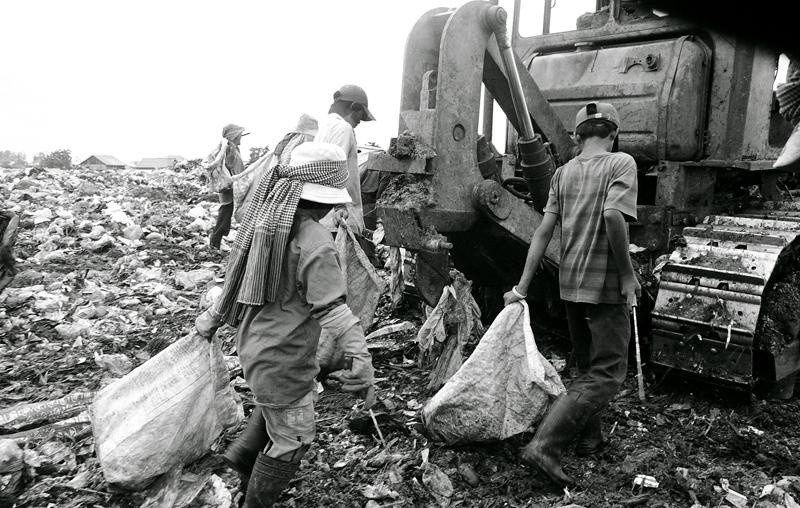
[
  {"x1": 511, "y1": 286, "x2": 528, "y2": 299},
  {"x1": 333, "y1": 203, "x2": 348, "y2": 211}
]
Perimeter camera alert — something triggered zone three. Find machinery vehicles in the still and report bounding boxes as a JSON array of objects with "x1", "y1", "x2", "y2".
[{"x1": 368, "y1": 1, "x2": 799, "y2": 398}]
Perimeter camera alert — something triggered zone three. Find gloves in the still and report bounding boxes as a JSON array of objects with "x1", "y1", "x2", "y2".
[
  {"x1": 191, "y1": 303, "x2": 227, "y2": 339},
  {"x1": 325, "y1": 322, "x2": 378, "y2": 412}
]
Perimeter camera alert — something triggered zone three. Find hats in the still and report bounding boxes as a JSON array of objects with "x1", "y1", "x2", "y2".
[
  {"x1": 574, "y1": 101, "x2": 621, "y2": 153},
  {"x1": 332, "y1": 83, "x2": 375, "y2": 124},
  {"x1": 287, "y1": 141, "x2": 356, "y2": 204}
]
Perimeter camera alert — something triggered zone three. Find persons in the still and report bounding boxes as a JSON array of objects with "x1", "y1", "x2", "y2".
[
  {"x1": 197, "y1": 143, "x2": 382, "y2": 508},
  {"x1": 500, "y1": 99, "x2": 645, "y2": 493},
  {"x1": 204, "y1": 124, "x2": 253, "y2": 255},
  {"x1": 308, "y1": 84, "x2": 375, "y2": 388},
  {"x1": 268, "y1": 113, "x2": 320, "y2": 173}
]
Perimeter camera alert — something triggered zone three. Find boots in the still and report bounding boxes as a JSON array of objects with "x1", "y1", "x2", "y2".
[
  {"x1": 218, "y1": 400, "x2": 268, "y2": 472},
  {"x1": 235, "y1": 449, "x2": 300, "y2": 507},
  {"x1": 518, "y1": 392, "x2": 590, "y2": 491},
  {"x1": 576, "y1": 407, "x2": 616, "y2": 455}
]
